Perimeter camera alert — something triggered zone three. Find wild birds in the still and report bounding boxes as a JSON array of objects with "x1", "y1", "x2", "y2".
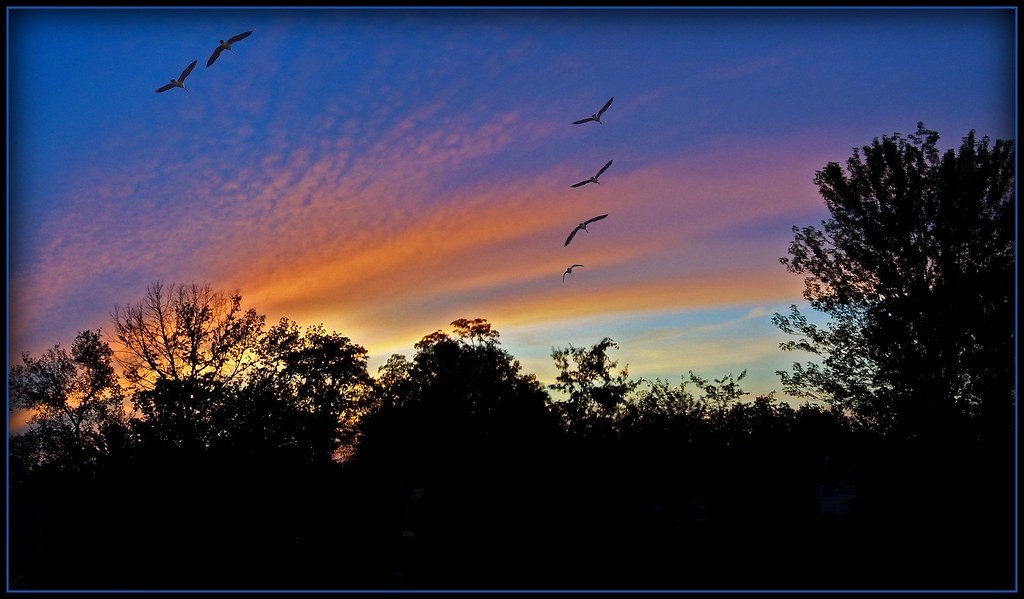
[
  {"x1": 155, "y1": 60, "x2": 198, "y2": 93},
  {"x1": 572, "y1": 96, "x2": 614, "y2": 125},
  {"x1": 563, "y1": 265, "x2": 584, "y2": 283},
  {"x1": 207, "y1": 31, "x2": 253, "y2": 67},
  {"x1": 569, "y1": 160, "x2": 613, "y2": 189},
  {"x1": 564, "y1": 214, "x2": 608, "y2": 247}
]
